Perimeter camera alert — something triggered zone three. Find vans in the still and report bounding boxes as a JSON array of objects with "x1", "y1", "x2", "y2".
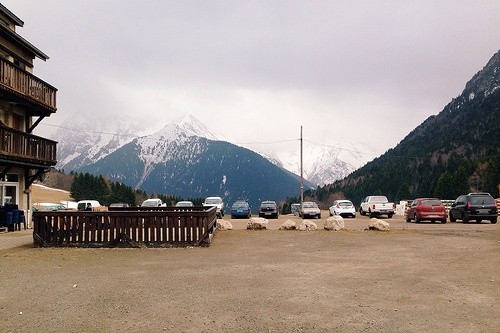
[{"x1": 76, "y1": 200, "x2": 103, "y2": 212}]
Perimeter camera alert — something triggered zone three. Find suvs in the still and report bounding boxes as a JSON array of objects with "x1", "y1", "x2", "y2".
[
  {"x1": 258, "y1": 200, "x2": 279, "y2": 219},
  {"x1": 202, "y1": 197, "x2": 225, "y2": 219},
  {"x1": 449, "y1": 192, "x2": 499, "y2": 224}
]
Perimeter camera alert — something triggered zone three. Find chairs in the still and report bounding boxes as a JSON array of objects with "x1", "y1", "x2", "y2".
[{"x1": 29, "y1": 86, "x2": 41, "y2": 97}]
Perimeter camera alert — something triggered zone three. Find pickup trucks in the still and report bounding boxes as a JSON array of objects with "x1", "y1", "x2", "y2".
[
  {"x1": 137, "y1": 198, "x2": 167, "y2": 215},
  {"x1": 359, "y1": 195, "x2": 396, "y2": 219}
]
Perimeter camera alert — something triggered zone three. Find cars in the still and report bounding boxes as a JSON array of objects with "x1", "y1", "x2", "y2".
[
  {"x1": 109, "y1": 203, "x2": 129, "y2": 207},
  {"x1": 173, "y1": 201, "x2": 195, "y2": 219},
  {"x1": 329, "y1": 200, "x2": 356, "y2": 219},
  {"x1": 230, "y1": 201, "x2": 252, "y2": 219},
  {"x1": 297, "y1": 202, "x2": 322, "y2": 219},
  {"x1": 405, "y1": 198, "x2": 448, "y2": 224}
]
